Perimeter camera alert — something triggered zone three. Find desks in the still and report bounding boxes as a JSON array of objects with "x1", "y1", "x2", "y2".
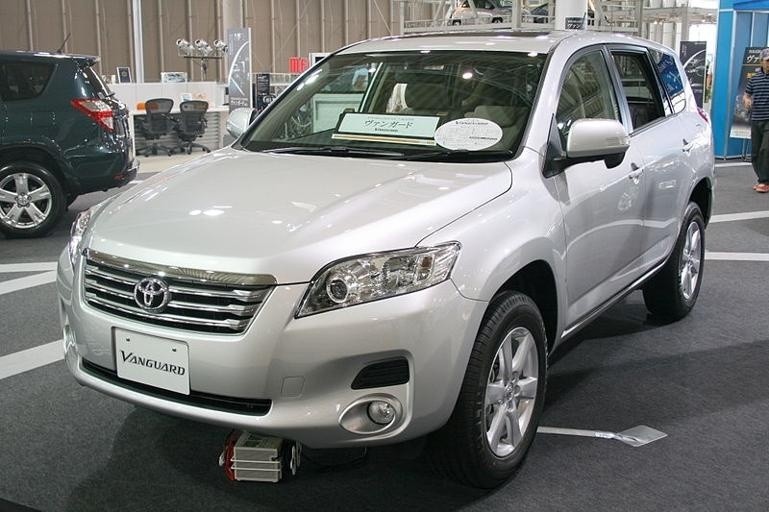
[{"x1": 126, "y1": 105, "x2": 229, "y2": 159}]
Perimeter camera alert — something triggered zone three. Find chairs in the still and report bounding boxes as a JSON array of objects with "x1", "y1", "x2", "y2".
[
  {"x1": 401, "y1": 79, "x2": 463, "y2": 130},
  {"x1": 172, "y1": 101, "x2": 211, "y2": 155},
  {"x1": 457, "y1": 104, "x2": 527, "y2": 152},
  {"x1": 136, "y1": 98, "x2": 175, "y2": 157}
]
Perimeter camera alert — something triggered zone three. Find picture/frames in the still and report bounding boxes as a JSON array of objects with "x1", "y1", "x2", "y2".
[{"x1": 116, "y1": 67, "x2": 132, "y2": 83}]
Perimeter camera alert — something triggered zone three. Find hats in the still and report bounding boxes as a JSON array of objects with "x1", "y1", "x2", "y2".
[{"x1": 760, "y1": 48, "x2": 769, "y2": 60}]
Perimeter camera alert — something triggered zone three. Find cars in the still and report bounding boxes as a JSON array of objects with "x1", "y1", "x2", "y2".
[
  {"x1": 1, "y1": 51, "x2": 141, "y2": 239},
  {"x1": 57, "y1": 23, "x2": 715, "y2": 491}
]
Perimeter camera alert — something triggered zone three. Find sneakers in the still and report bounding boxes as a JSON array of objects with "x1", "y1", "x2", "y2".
[{"x1": 752, "y1": 183, "x2": 769, "y2": 193}]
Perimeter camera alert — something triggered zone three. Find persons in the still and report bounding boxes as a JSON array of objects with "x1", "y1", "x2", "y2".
[
  {"x1": 706, "y1": 61, "x2": 715, "y2": 115},
  {"x1": 742, "y1": 47, "x2": 769, "y2": 194}
]
